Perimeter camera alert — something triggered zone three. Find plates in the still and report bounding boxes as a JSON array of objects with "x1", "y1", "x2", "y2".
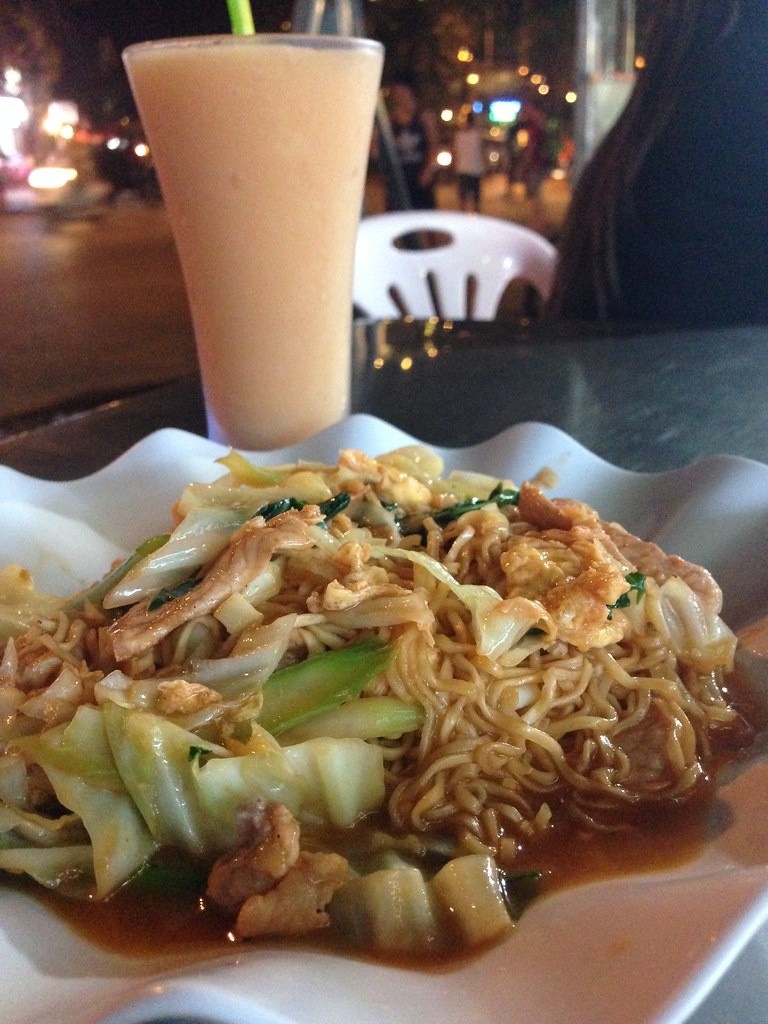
[{"x1": 0, "y1": 414, "x2": 768, "y2": 1024}]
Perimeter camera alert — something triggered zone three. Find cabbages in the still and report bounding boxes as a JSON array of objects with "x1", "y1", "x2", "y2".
[{"x1": 0, "y1": 443, "x2": 745, "y2": 959}]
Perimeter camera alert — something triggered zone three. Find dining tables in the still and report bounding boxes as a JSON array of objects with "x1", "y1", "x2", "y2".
[{"x1": 0, "y1": 320, "x2": 768, "y2": 1024}]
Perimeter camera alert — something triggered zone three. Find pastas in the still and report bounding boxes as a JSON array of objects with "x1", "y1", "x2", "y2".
[{"x1": 160, "y1": 523, "x2": 738, "y2": 859}]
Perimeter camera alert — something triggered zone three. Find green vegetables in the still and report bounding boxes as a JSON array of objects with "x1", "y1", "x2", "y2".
[
  {"x1": 201, "y1": 638, "x2": 400, "y2": 747},
  {"x1": 415, "y1": 479, "x2": 521, "y2": 540},
  {"x1": 252, "y1": 489, "x2": 352, "y2": 526}
]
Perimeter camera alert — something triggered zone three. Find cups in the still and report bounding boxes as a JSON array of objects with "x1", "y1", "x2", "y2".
[{"x1": 121, "y1": 34, "x2": 386, "y2": 452}]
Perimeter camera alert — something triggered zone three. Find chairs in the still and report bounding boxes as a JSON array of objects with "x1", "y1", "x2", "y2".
[{"x1": 354, "y1": 209, "x2": 558, "y2": 320}]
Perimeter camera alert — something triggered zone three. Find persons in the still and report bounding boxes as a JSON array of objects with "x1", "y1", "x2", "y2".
[
  {"x1": 453, "y1": 112, "x2": 490, "y2": 214},
  {"x1": 375, "y1": 83, "x2": 440, "y2": 212},
  {"x1": 501, "y1": 104, "x2": 551, "y2": 205},
  {"x1": 544, "y1": 0, "x2": 768, "y2": 321}
]
